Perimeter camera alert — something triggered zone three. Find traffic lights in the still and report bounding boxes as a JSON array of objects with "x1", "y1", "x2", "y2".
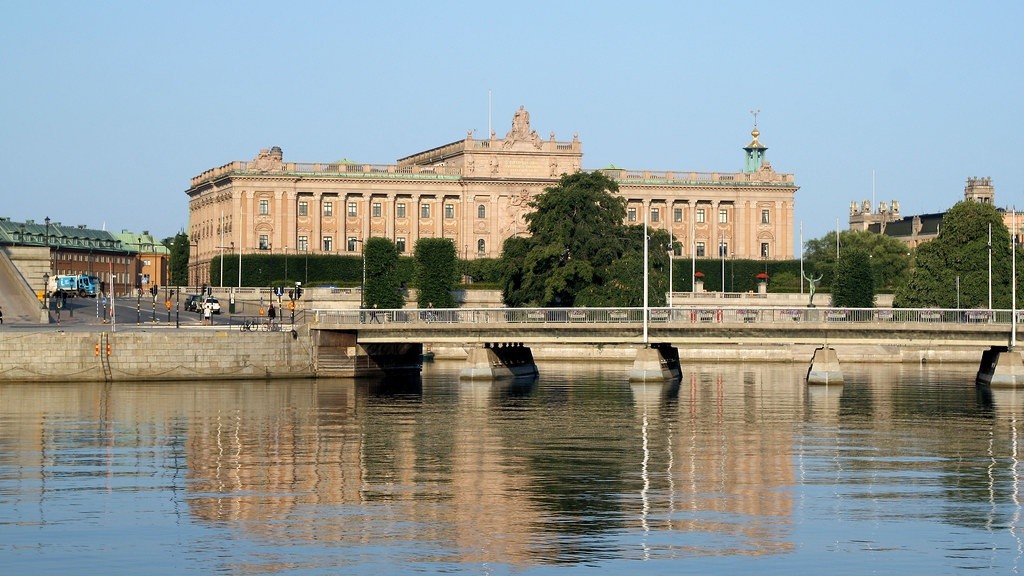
[
  {"x1": 288, "y1": 290, "x2": 293, "y2": 298},
  {"x1": 274, "y1": 283, "x2": 284, "y2": 296},
  {"x1": 297, "y1": 288, "x2": 302, "y2": 299},
  {"x1": 208, "y1": 287, "x2": 212, "y2": 296},
  {"x1": 150, "y1": 287, "x2": 153, "y2": 295},
  {"x1": 201, "y1": 283, "x2": 207, "y2": 295},
  {"x1": 169, "y1": 290, "x2": 174, "y2": 298}
]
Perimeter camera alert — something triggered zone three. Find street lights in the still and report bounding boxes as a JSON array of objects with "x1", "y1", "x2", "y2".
[
  {"x1": 45, "y1": 216, "x2": 50, "y2": 245},
  {"x1": 20, "y1": 223, "x2": 25, "y2": 243},
  {"x1": 731, "y1": 252, "x2": 734, "y2": 292},
  {"x1": 284, "y1": 245, "x2": 288, "y2": 289},
  {"x1": 170, "y1": 242, "x2": 198, "y2": 295},
  {"x1": 347, "y1": 237, "x2": 364, "y2": 323},
  {"x1": 39, "y1": 272, "x2": 50, "y2": 323},
  {"x1": 305, "y1": 242, "x2": 308, "y2": 287},
  {"x1": 55, "y1": 276, "x2": 59, "y2": 313},
  {"x1": 69, "y1": 278, "x2": 75, "y2": 317}
]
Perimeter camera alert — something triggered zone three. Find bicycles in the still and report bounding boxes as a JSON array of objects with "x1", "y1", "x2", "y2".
[
  {"x1": 261, "y1": 319, "x2": 278, "y2": 332},
  {"x1": 240, "y1": 319, "x2": 258, "y2": 332}
]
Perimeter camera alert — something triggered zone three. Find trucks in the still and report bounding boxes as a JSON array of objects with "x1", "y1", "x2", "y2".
[{"x1": 57, "y1": 276, "x2": 100, "y2": 298}]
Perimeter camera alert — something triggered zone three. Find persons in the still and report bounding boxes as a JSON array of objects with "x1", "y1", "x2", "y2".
[
  {"x1": 102, "y1": 296, "x2": 107, "y2": 305},
  {"x1": 512, "y1": 105, "x2": 530, "y2": 137},
  {"x1": 203, "y1": 306, "x2": 211, "y2": 326},
  {"x1": 426, "y1": 302, "x2": 435, "y2": 323},
  {"x1": 368, "y1": 304, "x2": 381, "y2": 324},
  {"x1": 268, "y1": 304, "x2": 275, "y2": 321}
]
